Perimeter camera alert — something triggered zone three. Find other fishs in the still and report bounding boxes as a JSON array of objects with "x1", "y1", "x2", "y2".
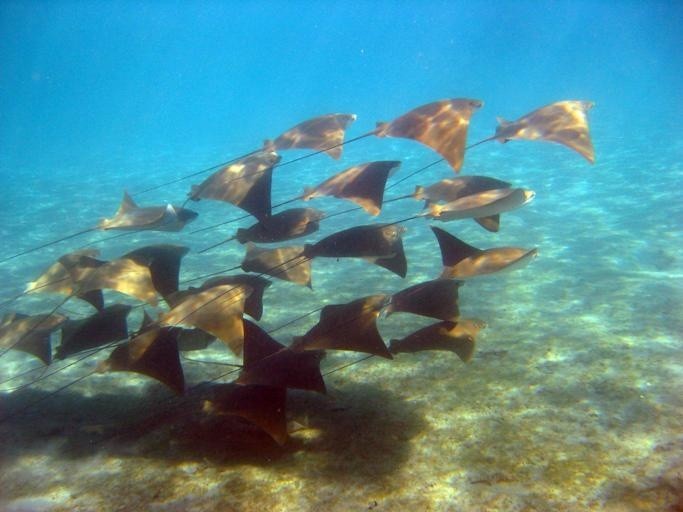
[{"x1": 1, "y1": 96, "x2": 595, "y2": 452}]
